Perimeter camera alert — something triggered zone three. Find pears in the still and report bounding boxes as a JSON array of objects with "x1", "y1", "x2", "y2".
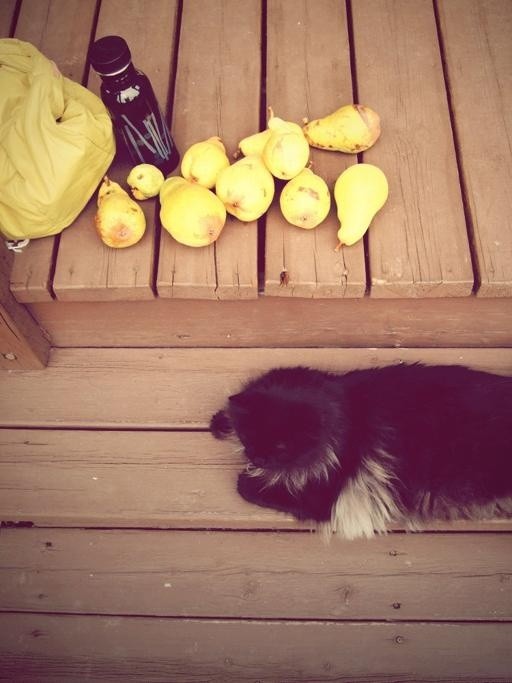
[{"x1": 95, "y1": 103, "x2": 390, "y2": 248}]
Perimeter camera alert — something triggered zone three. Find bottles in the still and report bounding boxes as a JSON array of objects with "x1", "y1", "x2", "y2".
[{"x1": 87, "y1": 34, "x2": 181, "y2": 178}]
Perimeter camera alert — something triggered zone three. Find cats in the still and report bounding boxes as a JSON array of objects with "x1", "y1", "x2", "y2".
[{"x1": 208, "y1": 356, "x2": 511, "y2": 547}]
